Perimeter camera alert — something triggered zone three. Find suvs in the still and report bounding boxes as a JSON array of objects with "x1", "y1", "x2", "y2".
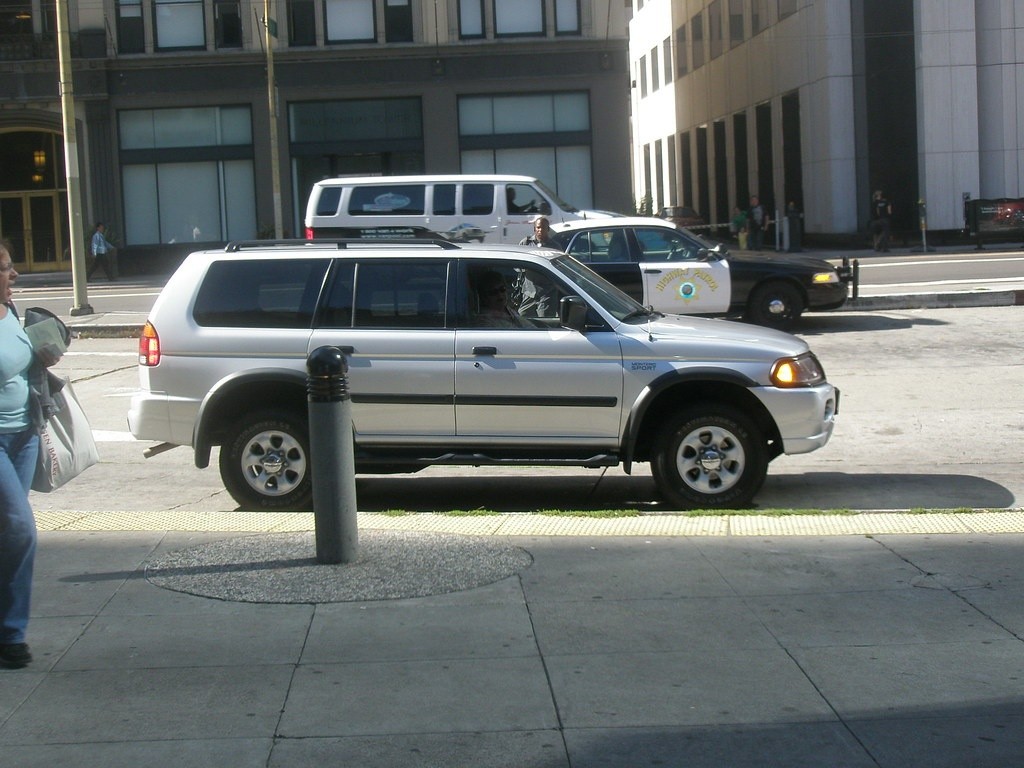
[{"x1": 128, "y1": 226, "x2": 840, "y2": 512}]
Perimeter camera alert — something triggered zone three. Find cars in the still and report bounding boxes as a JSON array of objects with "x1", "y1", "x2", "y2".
[
  {"x1": 654, "y1": 206, "x2": 706, "y2": 234},
  {"x1": 548, "y1": 217, "x2": 849, "y2": 329}
]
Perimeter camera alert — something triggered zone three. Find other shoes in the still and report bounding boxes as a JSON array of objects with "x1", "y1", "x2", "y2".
[{"x1": 0, "y1": 643, "x2": 32, "y2": 666}]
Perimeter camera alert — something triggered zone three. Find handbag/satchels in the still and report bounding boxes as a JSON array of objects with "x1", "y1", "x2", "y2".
[{"x1": 30, "y1": 369, "x2": 99, "y2": 493}]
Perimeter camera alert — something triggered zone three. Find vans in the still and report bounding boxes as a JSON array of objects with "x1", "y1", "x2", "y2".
[{"x1": 305, "y1": 174, "x2": 628, "y2": 245}]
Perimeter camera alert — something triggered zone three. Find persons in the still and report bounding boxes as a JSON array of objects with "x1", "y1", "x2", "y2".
[
  {"x1": 506, "y1": 187, "x2": 535, "y2": 213},
  {"x1": 746, "y1": 195, "x2": 769, "y2": 230},
  {"x1": 732, "y1": 206, "x2": 750, "y2": 250},
  {"x1": 475, "y1": 272, "x2": 537, "y2": 330},
  {"x1": 0, "y1": 239, "x2": 62, "y2": 666},
  {"x1": 86, "y1": 222, "x2": 117, "y2": 282},
  {"x1": 518, "y1": 217, "x2": 563, "y2": 318},
  {"x1": 869, "y1": 190, "x2": 892, "y2": 252}
]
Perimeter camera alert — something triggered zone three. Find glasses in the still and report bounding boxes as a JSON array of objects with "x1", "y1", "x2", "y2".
[
  {"x1": 0, "y1": 262, "x2": 17, "y2": 273},
  {"x1": 485, "y1": 285, "x2": 507, "y2": 295}
]
